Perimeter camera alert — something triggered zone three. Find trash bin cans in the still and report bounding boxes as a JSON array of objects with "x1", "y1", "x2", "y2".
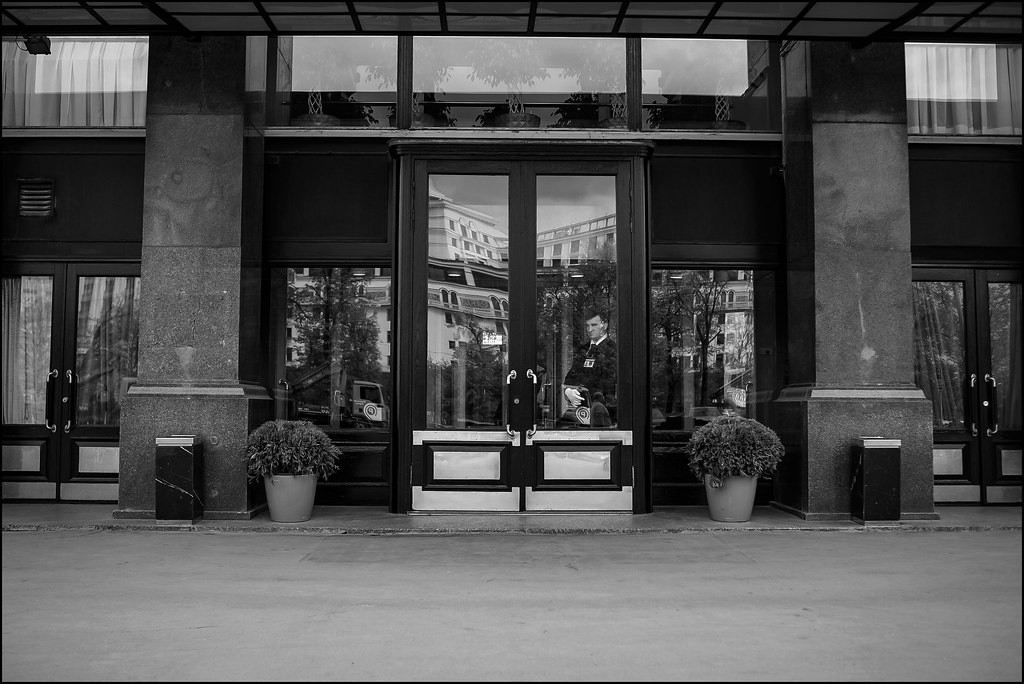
[
  {"x1": 849, "y1": 434, "x2": 902, "y2": 526},
  {"x1": 154, "y1": 434, "x2": 204, "y2": 526}
]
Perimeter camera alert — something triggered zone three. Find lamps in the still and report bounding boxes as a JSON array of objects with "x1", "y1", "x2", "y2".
[{"x1": 14, "y1": 31, "x2": 51, "y2": 55}]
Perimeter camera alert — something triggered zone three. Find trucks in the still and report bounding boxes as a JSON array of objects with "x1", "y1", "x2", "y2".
[{"x1": 299, "y1": 377, "x2": 389, "y2": 428}]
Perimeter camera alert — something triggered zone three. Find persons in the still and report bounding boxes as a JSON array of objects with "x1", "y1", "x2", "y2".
[{"x1": 564, "y1": 309, "x2": 617, "y2": 406}]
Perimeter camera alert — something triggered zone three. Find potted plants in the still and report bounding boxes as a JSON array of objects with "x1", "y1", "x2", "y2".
[
  {"x1": 685, "y1": 414, "x2": 785, "y2": 522},
  {"x1": 240, "y1": 418, "x2": 344, "y2": 522}
]
